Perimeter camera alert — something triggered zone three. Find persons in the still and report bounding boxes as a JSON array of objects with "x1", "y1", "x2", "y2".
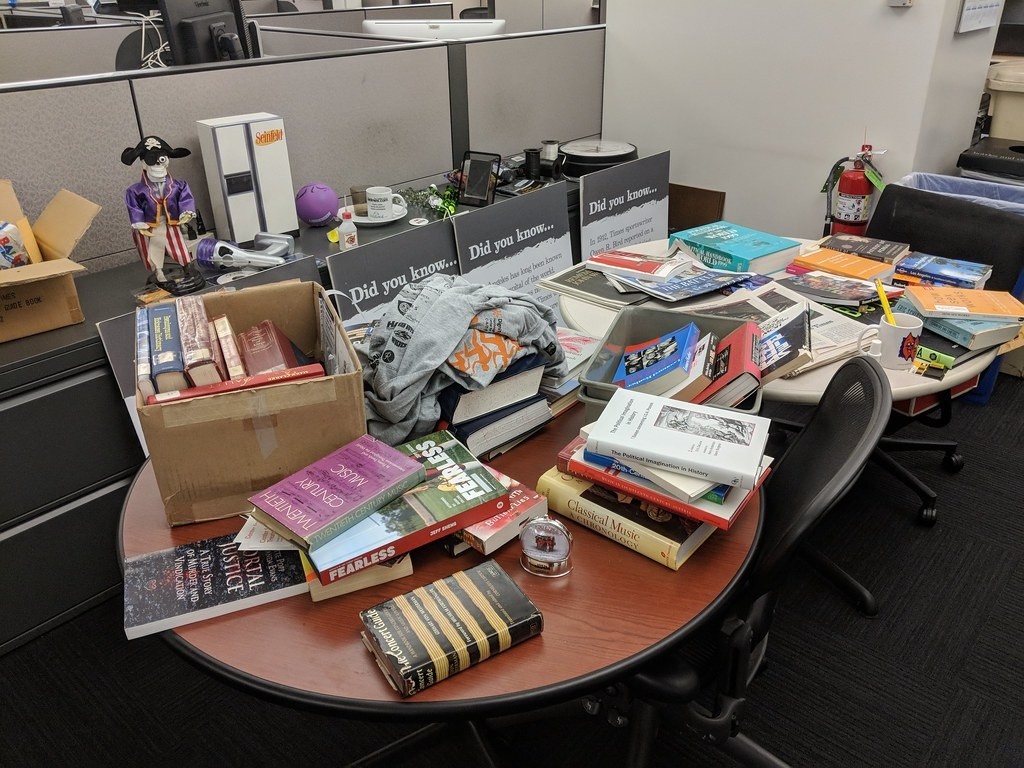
[
  {"x1": 626, "y1": 366, "x2": 637, "y2": 375},
  {"x1": 541, "y1": 219, "x2": 1024, "y2": 379},
  {"x1": 627, "y1": 360, "x2": 641, "y2": 365},
  {"x1": 645, "y1": 358, "x2": 659, "y2": 368},
  {"x1": 646, "y1": 353, "x2": 656, "y2": 359},
  {"x1": 644, "y1": 346, "x2": 657, "y2": 355},
  {"x1": 625, "y1": 354, "x2": 638, "y2": 361}
]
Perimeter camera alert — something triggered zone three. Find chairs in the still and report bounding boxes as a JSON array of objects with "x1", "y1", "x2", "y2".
[
  {"x1": 765, "y1": 184, "x2": 1024, "y2": 526},
  {"x1": 576, "y1": 356, "x2": 894, "y2": 766}
]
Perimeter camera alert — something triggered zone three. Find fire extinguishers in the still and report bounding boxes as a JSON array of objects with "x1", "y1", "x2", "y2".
[{"x1": 820, "y1": 145, "x2": 888, "y2": 238}]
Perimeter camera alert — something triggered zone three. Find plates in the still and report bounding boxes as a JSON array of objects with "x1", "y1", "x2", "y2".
[{"x1": 336, "y1": 204, "x2": 408, "y2": 226}]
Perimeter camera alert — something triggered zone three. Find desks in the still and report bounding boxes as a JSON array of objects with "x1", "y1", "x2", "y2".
[
  {"x1": 117, "y1": 400, "x2": 764, "y2": 767},
  {"x1": 560, "y1": 238, "x2": 1002, "y2": 620}
]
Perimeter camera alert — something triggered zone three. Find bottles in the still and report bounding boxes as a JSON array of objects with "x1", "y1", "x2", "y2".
[{"x1": 338, "y1": 211, "x2": 358, "y2": 251}]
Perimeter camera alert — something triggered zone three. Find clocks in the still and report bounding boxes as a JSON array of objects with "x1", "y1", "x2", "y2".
[{"x1": 558, "y1": 140, "x2": 638, "y2": 177}]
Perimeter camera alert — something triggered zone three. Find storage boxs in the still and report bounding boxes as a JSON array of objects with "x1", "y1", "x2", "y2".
[
  {"x1": 1, "y1": 177, "x2": 102, "y2": 343},
  {"x1": 196, "y1": 112, "x2": 299, "y2": 248},
  {"x1": 134, "y1": 281, "x2": 368, "y2": 527},
  {"x1": 576, "y1": 304, "x2": 766, "y2": 427}
]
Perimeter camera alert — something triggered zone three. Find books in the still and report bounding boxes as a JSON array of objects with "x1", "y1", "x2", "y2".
[
  {"x1": 135, "y1": 296, "x2": 323, "y2": 403},
  {"x1": 123, "y1": 531, "x2": 309, "y2": 641},
  {"x1": 437, "y1": 324, "x2": 604, "y2": 462},
  {"x1": 361, "y1": 559, "x2": 544, "y2": 698},
  {"x1": 536, "y1": 387, "x2": 774, "y2": 570},
  {"x1": 245, "y1": 428, "x2": 548, "y2": 601},
  {"x1": 613, "y1": 301, "x2": 812, "y2": 404}
]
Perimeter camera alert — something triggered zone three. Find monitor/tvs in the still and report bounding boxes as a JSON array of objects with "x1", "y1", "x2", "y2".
[
  {"x1": 60, "y1": 3, "x2": 85, "y2": 26},
  {"x1": 362, "y1": 18, "x2": 506, "y2": 40},
  {"x1": 158, "y1": 0, "x2": 254, "y2": 66}
]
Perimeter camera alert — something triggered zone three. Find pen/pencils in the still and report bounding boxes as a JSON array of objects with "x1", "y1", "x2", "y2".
[{"x1": 873, "y1": 277, "x2": 896, "y2": 325}]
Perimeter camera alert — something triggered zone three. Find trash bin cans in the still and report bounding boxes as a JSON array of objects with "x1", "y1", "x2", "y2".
[
  {"x1": 890, "y1": 172, "x2": 1024, "y2": 303},
  {"x1": 956, "y1": 137, "x2": 1024, "y2": 184},
  {"x1": 984, "y1": 60, "x2": 1024, "y2": 140}
]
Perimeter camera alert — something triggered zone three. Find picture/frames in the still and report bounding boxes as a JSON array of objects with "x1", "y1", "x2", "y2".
[{"x1": 457, "y1": 151, "x2": 501, "y2": 207}]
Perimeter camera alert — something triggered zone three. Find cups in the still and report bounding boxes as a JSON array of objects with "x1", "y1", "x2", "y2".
[
  {"x1": 857, "y1": 313, "x2": 923, "y2": 370},
  {"x1": 366, "y1": 186, "x2": 406, "y2": 221},
  {"x1": 350, "y1": 184, "x2": 374, "y2": 217}
]
